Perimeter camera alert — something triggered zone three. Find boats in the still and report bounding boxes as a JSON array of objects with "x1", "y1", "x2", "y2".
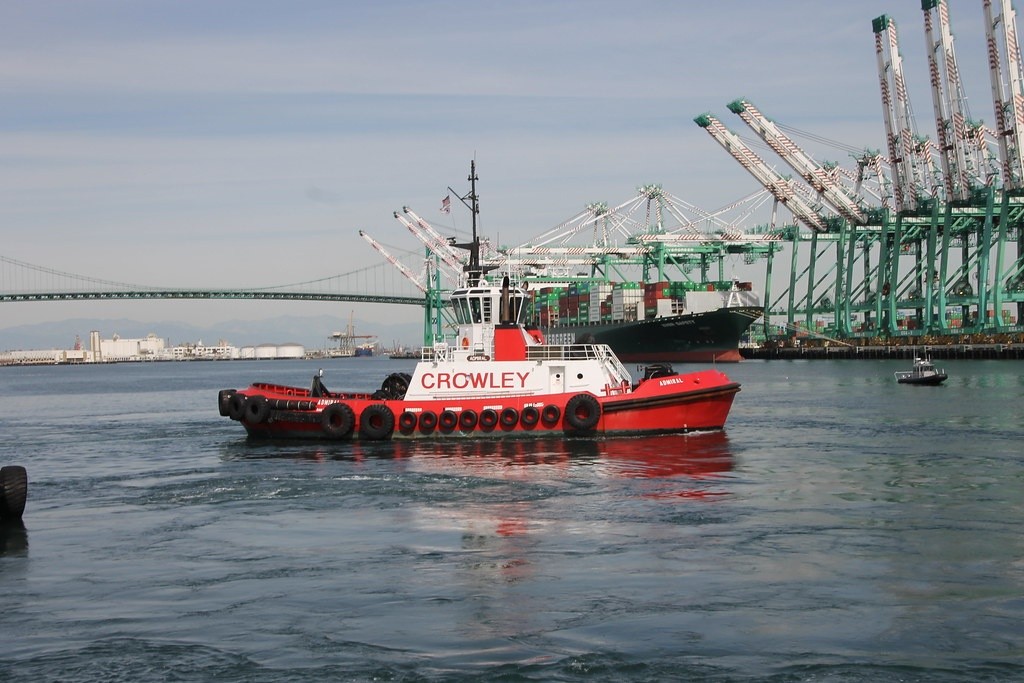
[
  {"x1": 218, "y1": 274, "x2": 742, "y2": 441},
  {"x1": 419, "y1": 147, "x2": 764, "y2": 364},
  {"x1": 894, "y1": 343, "x2": 949, "y2": 386}
]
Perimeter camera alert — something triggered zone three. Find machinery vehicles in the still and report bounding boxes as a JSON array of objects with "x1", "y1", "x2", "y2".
[{"x1": 356, "y1": 1, "x2": 1024, "y2": 346}]
